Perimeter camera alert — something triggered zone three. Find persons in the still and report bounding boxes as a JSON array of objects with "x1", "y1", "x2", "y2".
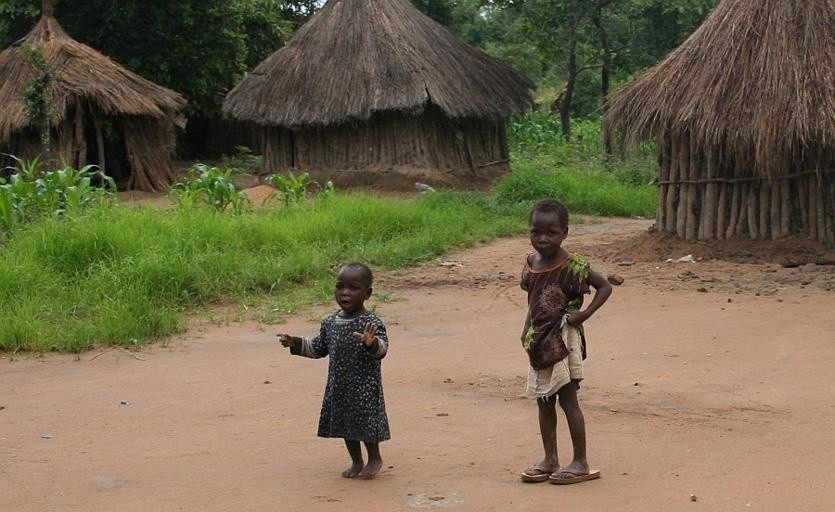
[
  {"x1": 517, "y1": 197, "x2": 614, "y2": 486},
  {"x1": 276, "y1": 261, "x2": 393, "y2": 479}
]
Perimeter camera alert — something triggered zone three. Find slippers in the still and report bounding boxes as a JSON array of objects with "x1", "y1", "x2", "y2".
[{"x1": 520, "y1": 464, "x2": 602, "y2": 486}]
